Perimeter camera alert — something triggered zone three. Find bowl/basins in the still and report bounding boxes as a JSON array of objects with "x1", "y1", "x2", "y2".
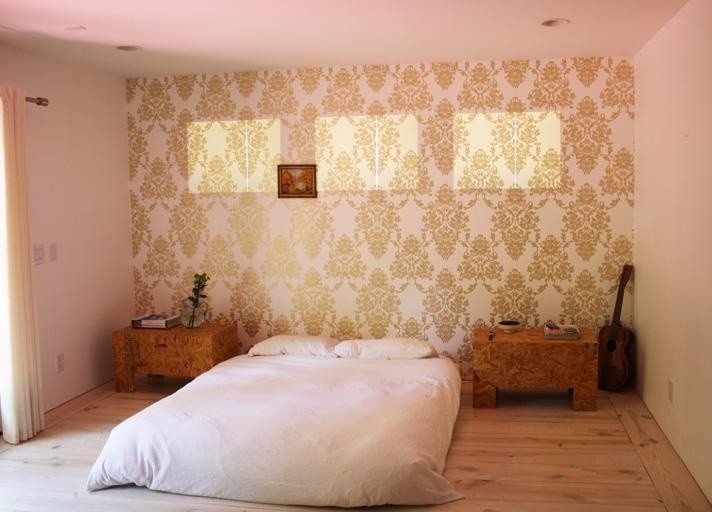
[{"x1": 499, "y1": 321, "x2": 521, "y2": 332}]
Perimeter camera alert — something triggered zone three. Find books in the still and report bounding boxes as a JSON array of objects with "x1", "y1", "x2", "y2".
[
  {"x1": 544, "y1": 325, "x2": 578, "y2": 339},
  {"x1": 131, "y1": 314, "x2": 181, "y2": 329}
]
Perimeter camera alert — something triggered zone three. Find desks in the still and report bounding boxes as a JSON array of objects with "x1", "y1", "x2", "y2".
[
  {"x1": 469, "y1": 326, "x2": 600, "y2": 410},
  {"x1": 112, "y1": 321, "x2": 238, "y2": 392}
]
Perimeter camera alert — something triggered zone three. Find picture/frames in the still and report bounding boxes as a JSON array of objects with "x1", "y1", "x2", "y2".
[{"x1": 276, "y1": 163, "x2": 318, "y2": 198}]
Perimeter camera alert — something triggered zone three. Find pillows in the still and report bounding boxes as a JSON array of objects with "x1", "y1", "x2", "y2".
[
  {"x1": 333, "y1": 337, "x2": 435, "y2": 360},
  {"x1": 247, "y1": 333, "x2": 341, "y2": 356}
]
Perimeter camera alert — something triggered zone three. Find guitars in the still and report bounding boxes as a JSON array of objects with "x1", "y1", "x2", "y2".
[{"x1": 598, "y1": 264, "x2": 635, "y2": 392}]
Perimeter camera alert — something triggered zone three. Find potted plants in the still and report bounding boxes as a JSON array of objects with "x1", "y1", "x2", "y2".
[{"x1": 178, "y1": 272, "x2": 212, "y2": 328}]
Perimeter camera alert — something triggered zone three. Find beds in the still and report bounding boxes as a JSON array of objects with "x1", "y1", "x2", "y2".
[{"x1": 89, "y1": 331, "x2": 465, "y2": 504}]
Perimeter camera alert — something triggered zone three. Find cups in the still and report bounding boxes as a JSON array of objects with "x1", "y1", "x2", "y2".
[{"x1": 218, "y1": 318, "x2": 225, "y2": 327}]
